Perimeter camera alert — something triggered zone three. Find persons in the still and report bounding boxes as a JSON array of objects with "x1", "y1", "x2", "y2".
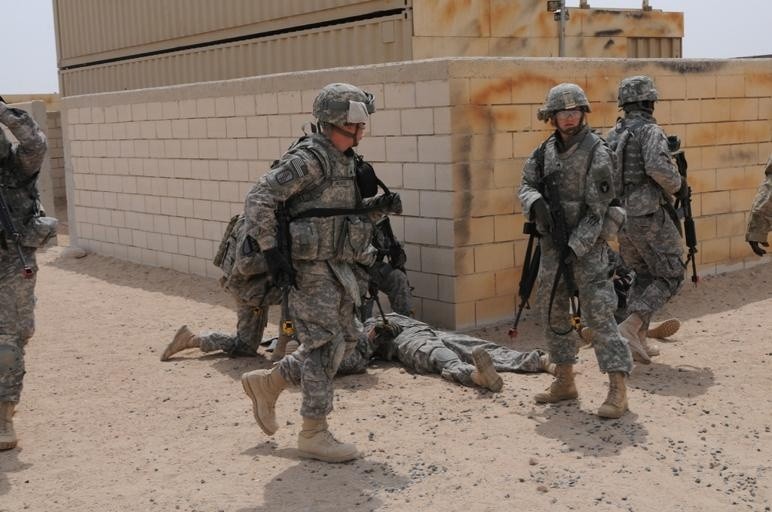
[
  {"x1": 569, "y1": 243, "x2": 679, "y2": 343},
  {"x1": 242, "y1": 82, "x2": 403, "y2": 464},
  {"x1": 363, "y1": 310, "x2": 558, "y2": 392},
  {"x1": 518, "y1": 83, "x2": 635, "y2": 420},
  {"x1": 745, "y1": 154, "x2": 772, "y2": 257},
  {"x1": 162, "y1": 137, "x2": 413, "y2": 362},
  {"x1": 0, "y1": 97, "x2": 47, "y2": 450},
  {"x1": 605, "y1": 76, "x2": 685, "y2": 365}
]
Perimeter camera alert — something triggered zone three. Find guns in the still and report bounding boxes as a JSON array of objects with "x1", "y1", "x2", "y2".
[
  {"x1": 0, "y1": 185, "x2": 31, "y2": 273},
  {"x1": 377, "y1": 217, "x2": 412, "y2": 294},
  {"x1": 271, "y1": 159, "x2": 290, "y2": 320},
  {"x1": 543, "y1": 171, "x2": 580, "y2": 325},
  {"x1": 669, "y1": 135, "x2": 698, "y2": 283},
  {"x1": 510, "y1": 217, "x2": 541, "y2": 328}
]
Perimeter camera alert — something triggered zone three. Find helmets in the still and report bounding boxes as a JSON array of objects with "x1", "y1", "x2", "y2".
[
  {"x1": 617, "y1": 75, "x2": 659, "y2": 107},
  {"x1": 537, "y1": 83, "x2": 593, "y2": 121},
  {"x1": 312, "y1": 82, "x2": 376, "y2": 129}
]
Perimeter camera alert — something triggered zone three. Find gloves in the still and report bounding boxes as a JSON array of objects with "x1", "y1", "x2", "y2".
[
  {"x1": 378, "y1": 192, "x2": 403, "y2": 216},
  {"x1": 749, "y1": 241, "x2": 769, "y2": 258},
  {"x1": 262, "y1": 247, "x2": 294, "y2": 277},
  {"x1": 532, "y1": 198, "x2": 554, "y2": 234},
  {"x1": 673, "y1": 176, "x2": 690, "y2": 202}
]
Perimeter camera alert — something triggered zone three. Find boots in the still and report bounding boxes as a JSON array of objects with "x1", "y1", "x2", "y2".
[
  {"x1": 638, "y1": 330, "x2": 660, "y2": 356},
  {"x1": 0, "y1": 402, "x2": 17, "y2": 450},
  {"x1": 534, "y1": 363, "x2": 578, "y2": 403},
  {"x1": 541, "y1": 351, "x2": 558, "y2": 376},
  {"x1": 160, "y1": 324, "x2": 200, "y2": 361},
  {"x1": 597, "y1": 371, "x2": 628, "y2": 419},
  {"x1": 648, "y1": 318, "x2": 681, "y2": 338},
  {"x1": 297, "y1": 417, "x2": 359, "y2": 463},
  {"x1": 470, "y1": 346, "x2": 504, "y2": 393},
  {"x1": 580, "y1": 326, "x2": 590, "y2": 345},
  {"x1": 617, "y1": 313, "x2": 652, "y2": 365},
  {"x1": 241, "y1": 368, "x2": 284, "y2": 436}
]
